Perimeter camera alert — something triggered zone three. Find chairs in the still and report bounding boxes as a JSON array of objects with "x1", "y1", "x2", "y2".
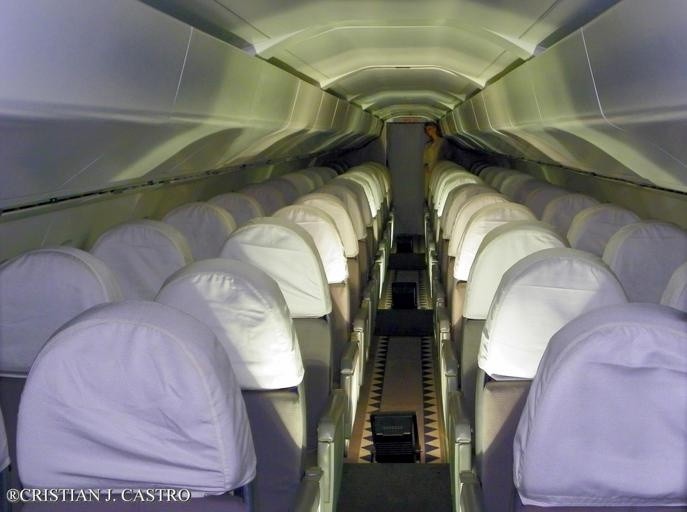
[
  {"x1": 423, "y1": 161, "x2": 687, "y2": 507},
  {"x1": 0, "y1": 161, "x2": 399, "y2": 512}
]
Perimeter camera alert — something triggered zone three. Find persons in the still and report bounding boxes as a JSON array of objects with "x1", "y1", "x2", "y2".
[{"x1": 422, "y1": 122, "x2": 446, "y2": 201}]
